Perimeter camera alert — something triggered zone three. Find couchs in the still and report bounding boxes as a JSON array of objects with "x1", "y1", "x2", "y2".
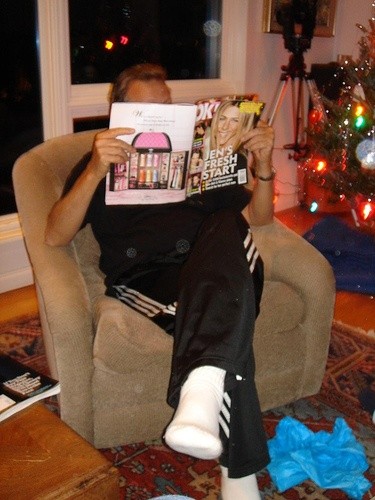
[{"x1": 11, "y1": 127, "x2": 338, "y2": 451}]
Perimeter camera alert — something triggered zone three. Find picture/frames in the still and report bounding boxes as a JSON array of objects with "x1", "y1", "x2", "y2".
[{"x1": 261, "y1": 0, "x2": 338, "y2": 38}]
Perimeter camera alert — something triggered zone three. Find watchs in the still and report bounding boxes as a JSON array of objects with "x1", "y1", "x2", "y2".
[{"x1": 258, "y1": 166, "x2": 276, "y2": 181}]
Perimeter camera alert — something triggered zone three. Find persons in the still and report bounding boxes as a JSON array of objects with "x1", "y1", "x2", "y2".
[
  {"x1": 204, "y1": 100, "x2": 248, "y2": 187},
  {"x1": 44, "y1": 64, "x2": 276, "y2": 500}
]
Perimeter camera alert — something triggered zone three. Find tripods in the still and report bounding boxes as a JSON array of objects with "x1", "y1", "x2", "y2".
[{"x1": 266, "y1": 54, "x2": 330, "y2": 161}]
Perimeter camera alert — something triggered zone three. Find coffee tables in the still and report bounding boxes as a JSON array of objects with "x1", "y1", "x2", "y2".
[{"x1": 0, "y1": 403, "x2": 122, "y2": 499}]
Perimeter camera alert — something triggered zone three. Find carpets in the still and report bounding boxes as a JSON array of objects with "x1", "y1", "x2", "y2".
[
  {"x1": 0, "y1": 306, "x2": 375, "y2": 500},
  {"x1": 301, "y1": 215, "x2": 375, "y2": 299}
]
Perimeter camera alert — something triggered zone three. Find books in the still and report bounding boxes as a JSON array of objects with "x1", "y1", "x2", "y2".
[
  {"x1": 0, "y1": 353, "x2": 61, "y2": 422},
  {"x1": 105, "y1": 94, "x2": 266, "y2": 205}
]
